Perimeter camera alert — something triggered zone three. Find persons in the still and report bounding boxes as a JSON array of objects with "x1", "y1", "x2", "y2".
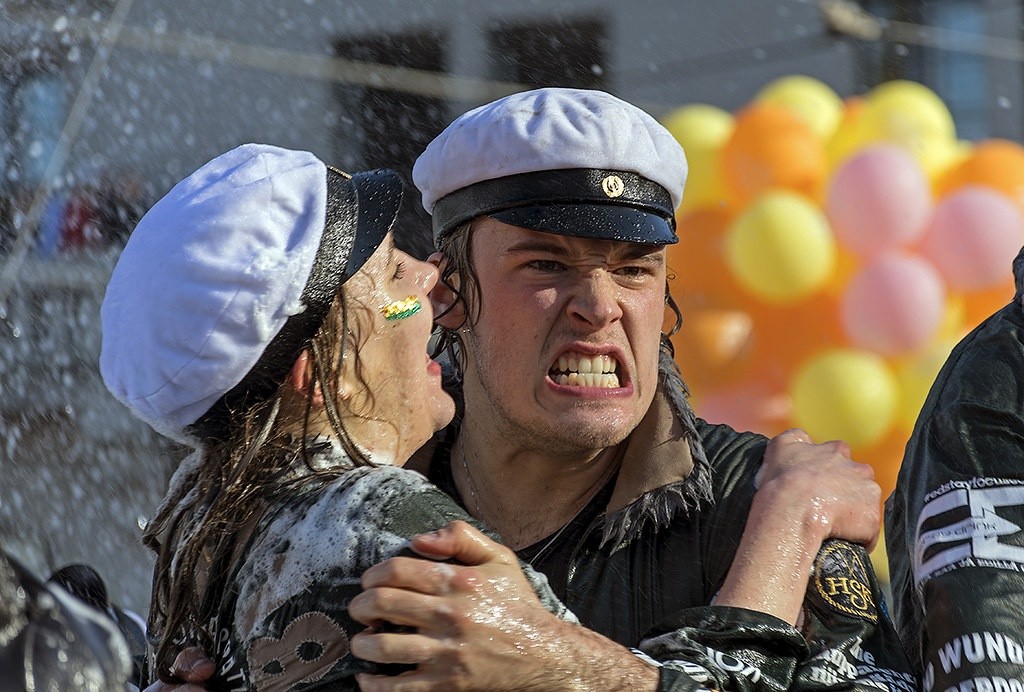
[
  {"x1": 879, "y1": 246, "x2": 1022, "y2": 690},
  {"x1": 336, "y1": 89, "x2": 928, "y2": 692},
  {"x1": 99, "y1": 146, "x2": 884, "y2": 692}
]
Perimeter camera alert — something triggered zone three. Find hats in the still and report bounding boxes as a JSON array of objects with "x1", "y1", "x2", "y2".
[
  {"x1": 98, "y1": 141, "x2": 407, "y2": 447},
  {"x1": 411, "y1": 86, "x2": 688, "y2": 245}
]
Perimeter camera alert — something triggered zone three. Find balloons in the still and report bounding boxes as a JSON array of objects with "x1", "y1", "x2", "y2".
[{"x1": 653, "y1": 75, "x2": 1024, "y2": 494}]
